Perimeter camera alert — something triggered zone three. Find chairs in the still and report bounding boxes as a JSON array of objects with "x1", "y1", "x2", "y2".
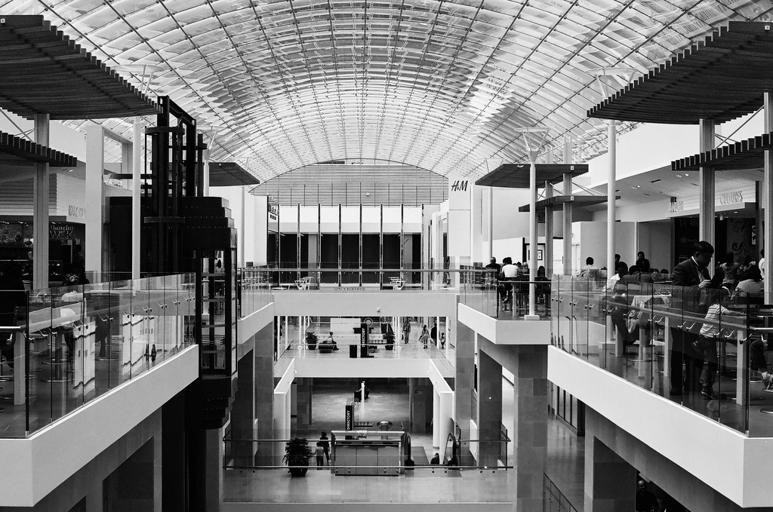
[
  {"x1": 518, "y1": 274, "x2": 548, "y2": 317},
  {"x1": 389, "y1": 267, "x2": 409, "y2": 292},
  {"x1": 296, "y1": 270, "x2": 319, "y2": 291}
]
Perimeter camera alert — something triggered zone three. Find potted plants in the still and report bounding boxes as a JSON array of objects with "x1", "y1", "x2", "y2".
[
  {"x1": 282, "y1": 438, "x2": 313, "y2": 478},
  {"x1": 306, "y1": 330, "x2": 317, "y2": 349}
]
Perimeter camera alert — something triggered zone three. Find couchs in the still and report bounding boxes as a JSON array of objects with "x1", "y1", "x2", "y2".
[{"x1": 319, "y1": 341, "x2": 335, "y2": 353}]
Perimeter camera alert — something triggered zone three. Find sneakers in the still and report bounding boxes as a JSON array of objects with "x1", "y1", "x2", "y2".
[{"x1": 702, "y1": 387, "x2": 716, "y2": 399}]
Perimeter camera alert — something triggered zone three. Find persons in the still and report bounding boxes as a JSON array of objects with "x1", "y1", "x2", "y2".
[
  {"x1": 440, "y1": 332, "x2": 445, "y2": 348},
  {"x1": 143, "y1": 344, "x2": 148, "y2": 367},
  {"x1": 429, "y1": 453, "x2": 439, "y2": 464},
  {"x1": 403, "y1": 318, "x2": 410, "y2": 344},
  {"x1": 319, "y1": 431, "x2": 329, "y2": 463},
  {"x1": 0, "y1": 265, "x2": 103, "y2": 411},
  {"x1": 721, "y1": 249, "x2": 773, "y2": 390},
  {"x1": 312, "y1": 443, "x2": 324, "y2": 469},
  {"x1": 430, "y1": 323, "x2": 437, "y2": 346},
  {"x1": 607, "y1": 251, "x2": 668, "y2": 344},
  {"x1": 149, "y1": 344, "x2": 157, "y2": 366},
  {"x1": 422, "y1": 325, "x2": 429, "y2": 349},
  {"x1": 486, "y1": 256, "x2": 528, "y2": 302},
  {"x1": 328, "y1": 332, "x2": 339, "y2": 351},
  {"x1": 671, "y1": 242, "x2": 744, "y2": 399},
  {"x1": 582, "y1": 256, "x2": 596, "y2": 272},
  {"x1": 535, "y1": 265, "x2": 549, "y2": 298}
]
